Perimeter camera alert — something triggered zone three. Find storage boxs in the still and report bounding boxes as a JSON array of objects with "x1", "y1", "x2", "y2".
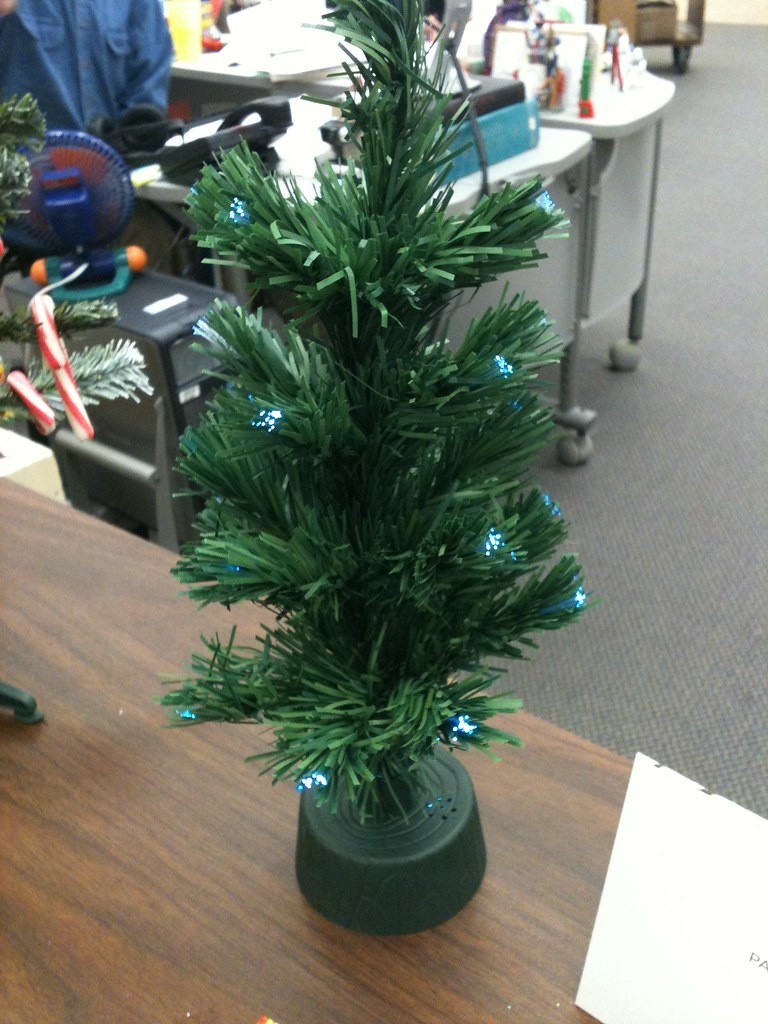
[
  {"x1": 593, "y1": 1, "x2": 638, "y2": 45},
  {"x1": 637, "y1": 1, "x2": 677, "y2": 39}
]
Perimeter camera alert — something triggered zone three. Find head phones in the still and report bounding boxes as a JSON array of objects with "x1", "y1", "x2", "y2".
[{"x1": 90, "y1": 105, "x2": 168, "y2": 154}]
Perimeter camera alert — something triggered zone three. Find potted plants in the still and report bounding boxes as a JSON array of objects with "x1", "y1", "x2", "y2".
[{"x1": 157, "y1": 0, "x2": 605, "y2": 935}]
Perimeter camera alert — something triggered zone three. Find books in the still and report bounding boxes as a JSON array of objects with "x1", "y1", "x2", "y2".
[{"x1": 326, "y1": 73, "x2": 540, "y2": 186}]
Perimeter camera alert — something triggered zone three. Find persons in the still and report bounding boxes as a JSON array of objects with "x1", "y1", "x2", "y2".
[
  {"x1": 0, "y1": 0, "x2": 183, "y2": 279},
  {"x1": 210, "y1": 0, "x2": 259, "y2": 41},
  {"x1": 326, "y1": 0, "x2": 458, "y2": 41}
]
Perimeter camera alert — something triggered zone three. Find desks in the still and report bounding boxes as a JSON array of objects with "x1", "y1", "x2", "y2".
[
  {"x1": 130, "y1": 0, "x2": 673, "y2": 466},
  {"x1": 0, "y1": 477, "x2": 623, "y2": 1024}
]
